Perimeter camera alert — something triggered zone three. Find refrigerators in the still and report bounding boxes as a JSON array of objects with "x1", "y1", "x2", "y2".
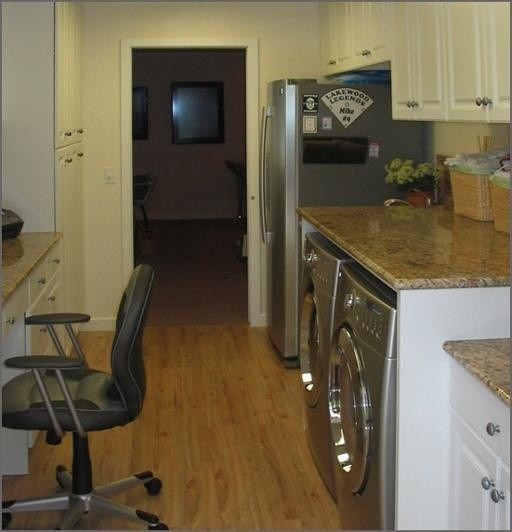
[{"x1": 257, "y1": 77, "x2": 434, "y2": 366}]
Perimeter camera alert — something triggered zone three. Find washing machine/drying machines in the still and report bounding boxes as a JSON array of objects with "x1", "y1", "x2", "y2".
[
  {"x1": 298, "y1": 232, "x2": 352, "y2": 502},
  {"x1": 326, "y1": 261, "x2": 397, "y2": 532}
]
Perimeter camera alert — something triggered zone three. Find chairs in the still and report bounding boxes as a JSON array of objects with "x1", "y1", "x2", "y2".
[{"x1": 0, "y1": 263, "x2": 170, "y2": 532}]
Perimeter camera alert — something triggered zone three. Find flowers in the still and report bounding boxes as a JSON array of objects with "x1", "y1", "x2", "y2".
[{"x1": 383, "y1": 156, "x2": 441, "y2": 189}]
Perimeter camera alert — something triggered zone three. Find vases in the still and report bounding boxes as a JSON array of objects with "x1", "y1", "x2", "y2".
[{"x1": 407, "y1": 185, "x2": 434, "y2": 206}]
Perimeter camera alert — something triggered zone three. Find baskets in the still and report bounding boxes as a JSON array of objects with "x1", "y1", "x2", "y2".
[
  {"x1": 489, "y1": 180, "x2": 512, "y2": 233},
  {"x1": 449, "y1": 170, "x2": 493, "y2": 221}
]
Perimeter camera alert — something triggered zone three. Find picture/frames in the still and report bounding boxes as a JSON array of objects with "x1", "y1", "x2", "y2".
[
  {"x1": 169, "y1": 81, "x2": 227, "y2": 145},
  {"x1": 132, "y1": 86, "x2": 150, "y2": 140}
]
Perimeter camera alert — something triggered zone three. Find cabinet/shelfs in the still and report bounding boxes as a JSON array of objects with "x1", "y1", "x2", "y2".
[
  {"x1": 447, "y1": 358, "x2": 510, "y2": 532},
  {"x1": 391, "y1": 3, "x2": 510, "y2": 124},
  {"x1": 316, "y1": 1, "x2": 391, "y2": 78}
]
